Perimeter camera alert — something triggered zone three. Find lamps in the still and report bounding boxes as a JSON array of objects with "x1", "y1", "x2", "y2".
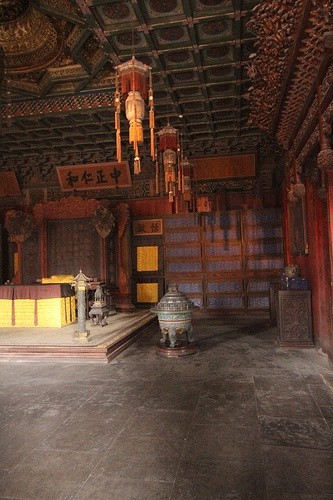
[
  {"x1": 112, "y1": 16, "x2": 156, "y2": 176},
  {"x1": 155, "y1": 105, "x2": 184, "y2": 202}
]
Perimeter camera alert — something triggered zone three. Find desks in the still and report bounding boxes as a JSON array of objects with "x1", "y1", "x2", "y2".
[{"x1": 0, "y1": 283, "x2": 76, "y2": 327}]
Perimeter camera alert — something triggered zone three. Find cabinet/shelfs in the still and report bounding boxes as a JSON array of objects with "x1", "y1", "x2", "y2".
[{"x1": 268, "y1": 281, "x2": 315, "y2": 349}]
